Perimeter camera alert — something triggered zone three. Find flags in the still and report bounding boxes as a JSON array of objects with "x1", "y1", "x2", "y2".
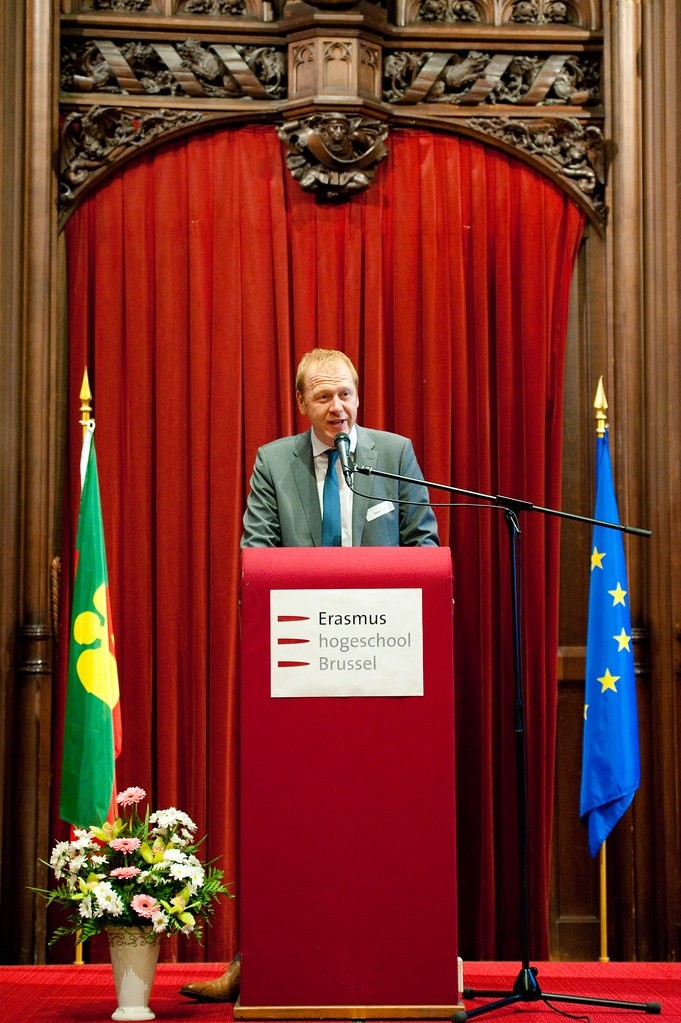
[
  {"x1": 580, "y1": 428, "x2": 641, "y2": 860},
  {"x1": 58, "y1": 422, "x2": 124, "y2": 867}
]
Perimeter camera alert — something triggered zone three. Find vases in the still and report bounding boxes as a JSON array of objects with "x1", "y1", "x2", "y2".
[{"x1": 105, "y1": 918, "x2": 161, "y2": 1022}]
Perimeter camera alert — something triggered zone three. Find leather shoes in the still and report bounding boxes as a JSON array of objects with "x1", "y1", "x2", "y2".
[{"x1": 179, "y1": 951, "x2": 240, "y2": 1003}]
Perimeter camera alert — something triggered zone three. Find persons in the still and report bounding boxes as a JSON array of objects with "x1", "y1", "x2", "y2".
[
  {"x1": 314, "y1": 112, "x2": 357, "y2": 162},
  {"x1": 179, "y1": 347, "x2": 442, "y2": 1003}
]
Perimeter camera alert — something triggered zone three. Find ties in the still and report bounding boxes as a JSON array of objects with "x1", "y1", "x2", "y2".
[{"x1": 321, "y1": 449, "x2": 342, "y2": 546}]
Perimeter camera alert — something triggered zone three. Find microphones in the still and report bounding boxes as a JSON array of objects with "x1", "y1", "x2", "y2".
[{"x1": 334, "y1": 431, "x2": 355, "y2": 487}]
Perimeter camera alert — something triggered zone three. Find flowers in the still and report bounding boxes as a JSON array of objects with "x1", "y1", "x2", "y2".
[{"x1": 31, "y1": 781, "x2": 230, "y2": 958}]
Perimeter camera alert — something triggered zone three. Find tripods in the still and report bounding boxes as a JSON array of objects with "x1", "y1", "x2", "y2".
[{"x1": 452, "y1": 508, "x2": 662, "y2": 1022}]
